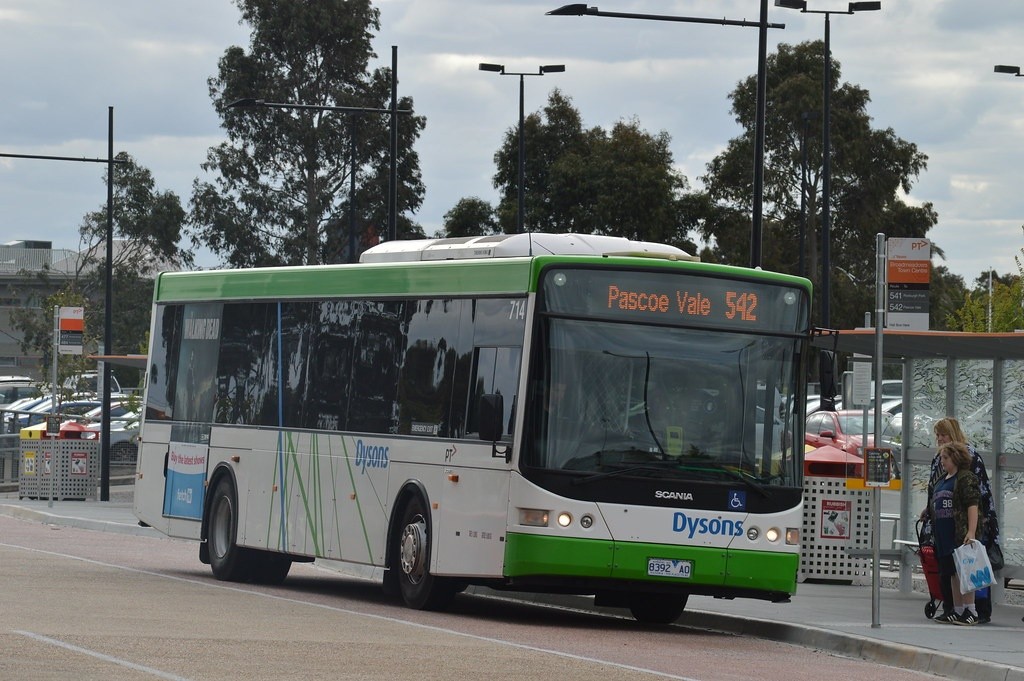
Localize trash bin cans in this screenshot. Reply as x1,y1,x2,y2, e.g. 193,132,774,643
19,420,48,500
39,421,99,501
795,444,873,586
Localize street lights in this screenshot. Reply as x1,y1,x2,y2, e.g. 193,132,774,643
770,0,884,351
546,0,784,270
479,62,570,235
226,41,417,244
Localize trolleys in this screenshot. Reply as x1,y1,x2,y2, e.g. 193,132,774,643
915,513,952,618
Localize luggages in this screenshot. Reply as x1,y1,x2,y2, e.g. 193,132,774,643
916,516,944,618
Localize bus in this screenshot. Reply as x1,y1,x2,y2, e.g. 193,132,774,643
132,232,845,628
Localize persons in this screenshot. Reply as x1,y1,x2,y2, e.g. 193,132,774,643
921,417,1001,623
920,442,981,626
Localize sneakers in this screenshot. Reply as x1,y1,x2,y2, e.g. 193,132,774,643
946,609,978,627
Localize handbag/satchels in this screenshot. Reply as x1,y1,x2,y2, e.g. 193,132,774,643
956,538,998,594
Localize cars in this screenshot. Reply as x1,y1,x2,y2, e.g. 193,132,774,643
1,368,144,464
746,376,904,472
